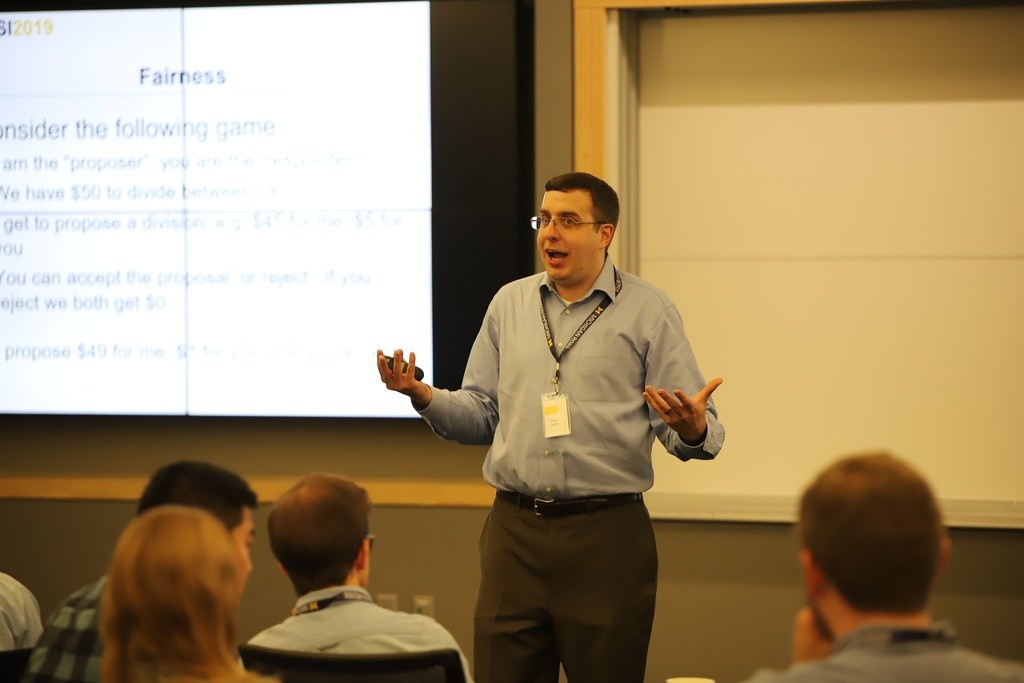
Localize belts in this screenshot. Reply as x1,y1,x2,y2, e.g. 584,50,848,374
495,488,643,518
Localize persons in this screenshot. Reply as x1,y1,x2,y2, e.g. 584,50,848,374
239,472,472,683
99,503,281,683
24,462,256,683
746,453,1023,683
376,170,724,683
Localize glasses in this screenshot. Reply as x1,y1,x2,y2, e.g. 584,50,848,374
530,215,607,231
367,534,376,551
